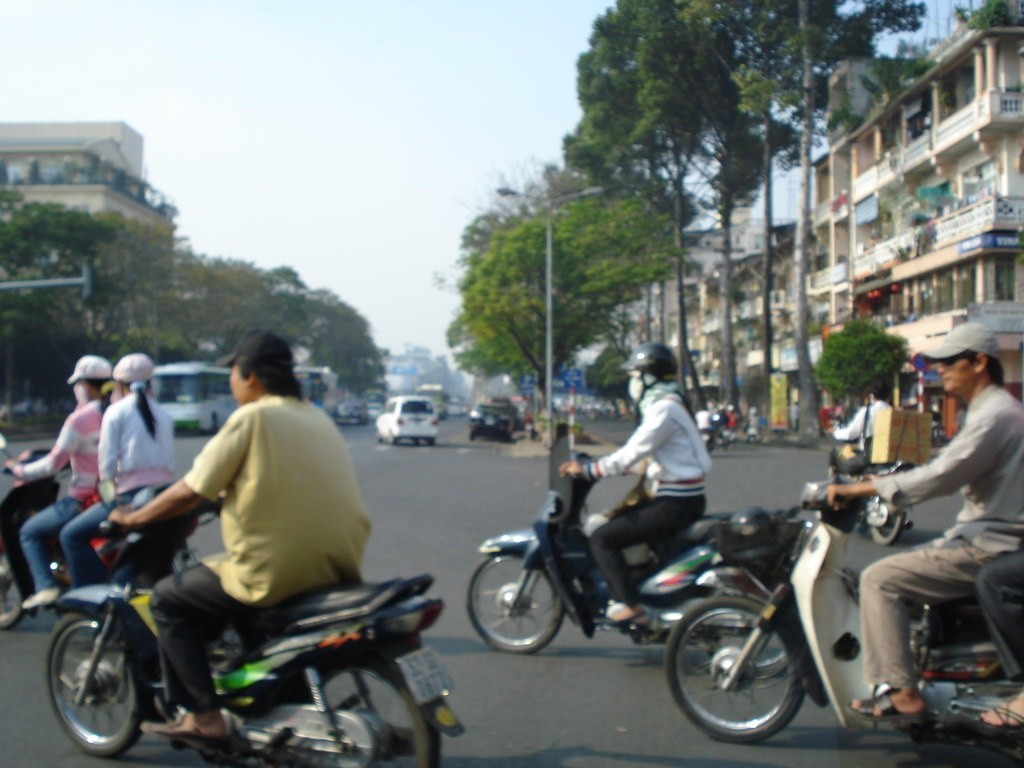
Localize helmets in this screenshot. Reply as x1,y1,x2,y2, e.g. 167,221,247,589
618,342,677,375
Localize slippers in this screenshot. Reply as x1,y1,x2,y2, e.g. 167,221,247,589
845,688,927,724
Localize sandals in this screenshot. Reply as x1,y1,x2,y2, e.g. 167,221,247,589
980,700,1024,734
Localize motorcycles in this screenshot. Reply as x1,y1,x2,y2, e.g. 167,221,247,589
41,479,468,768
463,451,801,679
660,444,1024,759
0,430,193,635
821,410,913,547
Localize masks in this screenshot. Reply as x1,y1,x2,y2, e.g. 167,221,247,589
72,384,88,403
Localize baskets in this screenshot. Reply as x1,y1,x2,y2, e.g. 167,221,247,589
712,511,805,570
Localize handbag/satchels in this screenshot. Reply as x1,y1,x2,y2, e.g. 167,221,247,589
835,443,868,475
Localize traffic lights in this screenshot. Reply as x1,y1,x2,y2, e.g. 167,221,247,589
79,260,108,309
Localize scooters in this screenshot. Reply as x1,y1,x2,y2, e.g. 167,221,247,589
700,410,762,452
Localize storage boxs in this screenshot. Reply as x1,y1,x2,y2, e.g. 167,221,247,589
870,408,932,465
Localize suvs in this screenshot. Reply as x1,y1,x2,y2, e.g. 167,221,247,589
373,395,438,446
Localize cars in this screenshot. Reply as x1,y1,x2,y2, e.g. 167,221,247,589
465,400,515,441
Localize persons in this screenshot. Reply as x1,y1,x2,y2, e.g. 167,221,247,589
108,332,373,746
694,399,763,444
832,381,892,477
976,550,1024,731
794,402,800,432
829,322,1024,720
4,351,176,610
558,342,708,627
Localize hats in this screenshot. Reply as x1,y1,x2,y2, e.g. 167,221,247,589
113,353,153,383
67,355,112,384
924,321,1000,361
215,329,295,369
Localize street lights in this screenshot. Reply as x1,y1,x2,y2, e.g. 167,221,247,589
496,184,606,451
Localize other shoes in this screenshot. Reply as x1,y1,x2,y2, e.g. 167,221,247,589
20,587,60,609
141,713,232,752
592,599,645,625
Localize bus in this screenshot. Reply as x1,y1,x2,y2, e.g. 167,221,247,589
147,361,239,436
338,382,468,426
294,365,339,418
